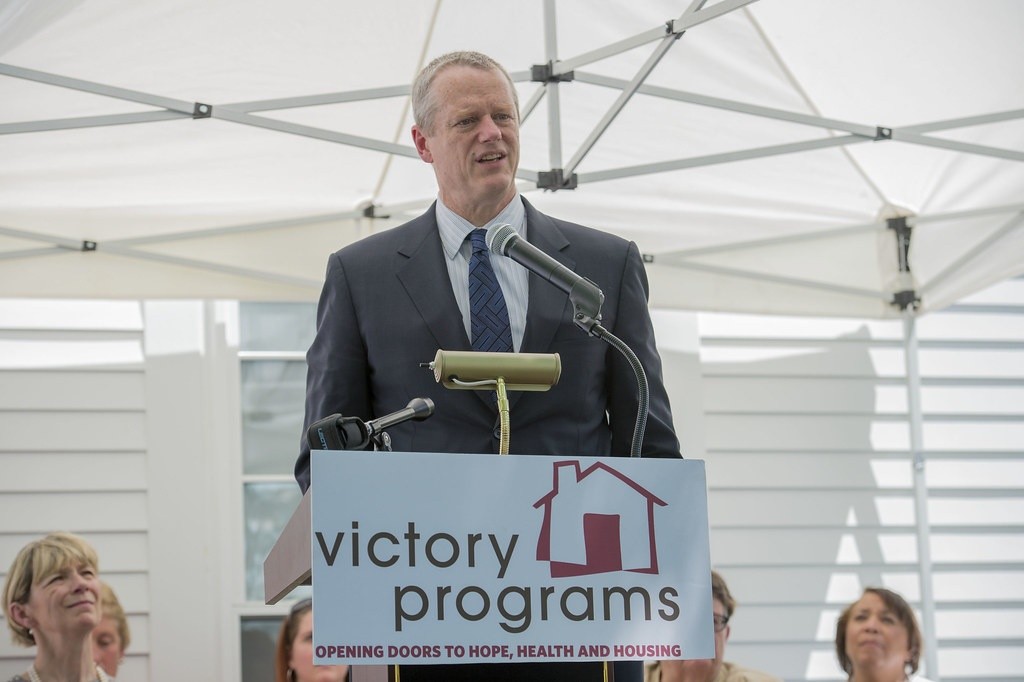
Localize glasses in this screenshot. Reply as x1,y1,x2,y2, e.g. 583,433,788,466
713,612,730,632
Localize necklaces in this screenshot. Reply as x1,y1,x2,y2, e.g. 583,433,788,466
26,662,109,682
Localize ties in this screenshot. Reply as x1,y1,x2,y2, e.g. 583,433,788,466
464,229,515,408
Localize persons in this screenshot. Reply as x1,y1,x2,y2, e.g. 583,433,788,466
91,582,130,677
834,588,934,682
644,570,779,682
275,597,354,682
293,50,686,498
0,533,132,682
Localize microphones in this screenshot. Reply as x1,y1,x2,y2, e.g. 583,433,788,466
307,397,437,451
485,222,605,311
